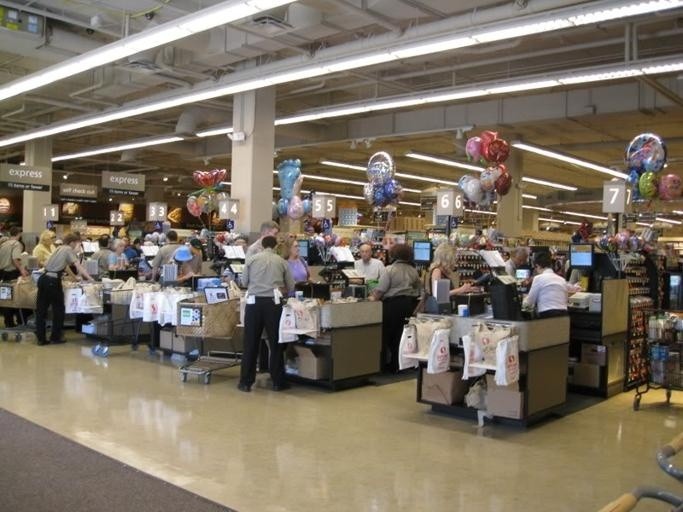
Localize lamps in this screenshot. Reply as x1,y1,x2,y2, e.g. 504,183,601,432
220,140,683,228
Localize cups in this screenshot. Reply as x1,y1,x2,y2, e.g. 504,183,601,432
294,290,303,300
457,304,467,317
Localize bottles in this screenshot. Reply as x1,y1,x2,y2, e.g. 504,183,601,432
648,313,665,339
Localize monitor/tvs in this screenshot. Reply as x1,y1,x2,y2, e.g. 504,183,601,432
569,243,594,269
413,241,431,263
297,240,309,259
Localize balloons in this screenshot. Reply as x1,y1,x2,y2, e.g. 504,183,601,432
273,158,311,220
187,168,230,219
363,151,403,208
458,130,512,209
571,132,682,272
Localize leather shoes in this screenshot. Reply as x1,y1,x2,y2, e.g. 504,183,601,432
272,381,293,392
50,338,67,345
38,340,51,346
237,383,251,392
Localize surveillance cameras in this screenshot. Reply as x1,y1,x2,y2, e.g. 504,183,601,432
145,12,154,20
87,28,94,35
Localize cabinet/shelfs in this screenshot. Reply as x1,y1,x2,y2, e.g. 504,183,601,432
327,242,669,392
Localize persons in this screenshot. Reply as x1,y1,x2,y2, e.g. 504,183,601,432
35,234,94,346
175,239,202,285
503,248,569,317
428,243,481,299
86,234,143,279
0,223,56,328
241,236,291,391
151,231,185,281
368,244,420,377
246,222,309,296
354,243,385,295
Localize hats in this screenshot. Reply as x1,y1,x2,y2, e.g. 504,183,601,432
190,238,203,249
166,230,178,242
175,246,193,262
391,244,413,260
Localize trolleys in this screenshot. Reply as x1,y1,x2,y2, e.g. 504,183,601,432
176,288,260,384
0,270,54,343
80,275,156,358
632,308,682,410
